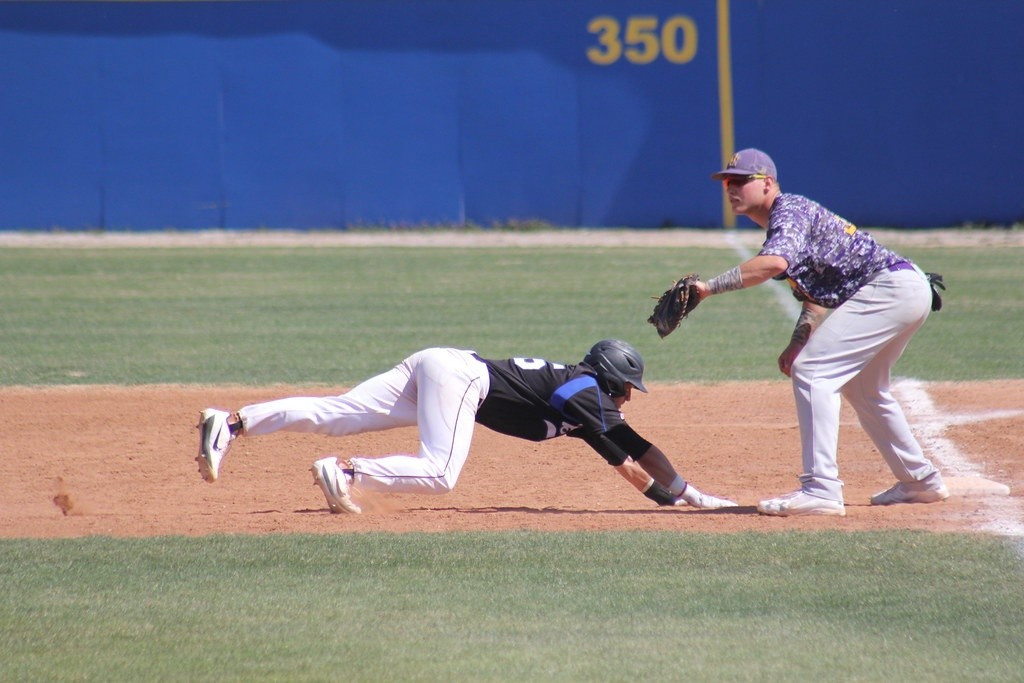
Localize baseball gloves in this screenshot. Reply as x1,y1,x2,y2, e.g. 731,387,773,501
647,273,699,339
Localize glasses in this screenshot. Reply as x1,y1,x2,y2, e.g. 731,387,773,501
723,173,767,189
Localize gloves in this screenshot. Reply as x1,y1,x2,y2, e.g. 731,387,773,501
926,271,946,312
679,482,740,509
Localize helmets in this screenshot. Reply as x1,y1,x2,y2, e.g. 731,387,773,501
584,339,649,399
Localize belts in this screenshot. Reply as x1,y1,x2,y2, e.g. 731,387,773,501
860,263,915,287
472,353,490,405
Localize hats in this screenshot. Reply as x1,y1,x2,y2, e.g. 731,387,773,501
711,147,777,181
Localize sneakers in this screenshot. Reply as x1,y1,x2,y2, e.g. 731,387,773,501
758,481,847,517
195,408,236,484
308,457,363,516
869,471,950,505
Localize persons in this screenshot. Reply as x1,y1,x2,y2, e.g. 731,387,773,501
646,148,950,516
195,340,740,515
